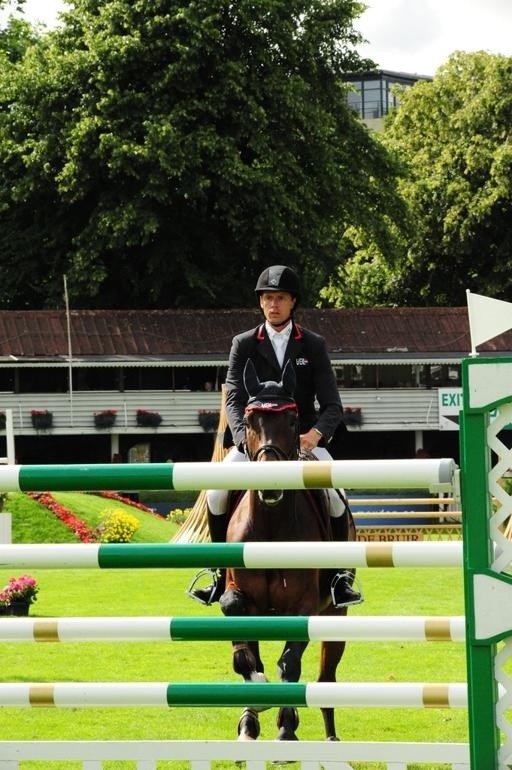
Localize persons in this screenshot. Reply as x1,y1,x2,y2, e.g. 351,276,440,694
192,265,362,602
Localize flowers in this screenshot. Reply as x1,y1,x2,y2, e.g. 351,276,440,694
341,406,365,431
0,573,41,606
0,407,220,439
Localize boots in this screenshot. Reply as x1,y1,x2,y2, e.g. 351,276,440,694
330,509,365,609
191,502,231,604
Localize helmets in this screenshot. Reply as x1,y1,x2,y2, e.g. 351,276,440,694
254,266,300,293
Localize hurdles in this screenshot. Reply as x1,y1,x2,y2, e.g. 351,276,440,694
0,357,511,769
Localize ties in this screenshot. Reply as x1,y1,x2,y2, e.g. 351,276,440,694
272,334,286,370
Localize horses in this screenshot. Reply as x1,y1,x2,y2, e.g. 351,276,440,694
220,356,358,767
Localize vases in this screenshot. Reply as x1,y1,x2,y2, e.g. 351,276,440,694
0,603,29,617
